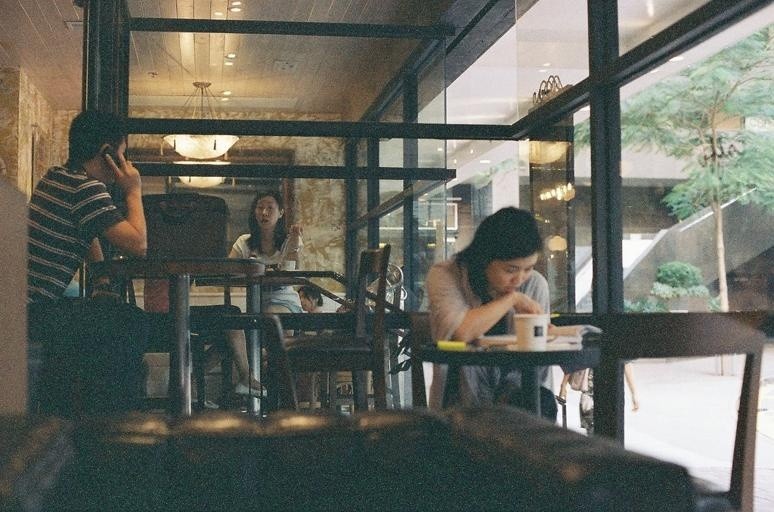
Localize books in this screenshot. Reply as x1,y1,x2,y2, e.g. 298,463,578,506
476,324,602,353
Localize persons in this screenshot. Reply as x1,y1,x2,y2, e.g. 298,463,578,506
28,109,146,413
425,206,558,425
558,361,640,438
189,190,301,416
297,286,332,336
143,195,266,409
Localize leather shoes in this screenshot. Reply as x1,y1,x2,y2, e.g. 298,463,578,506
234,381,268,400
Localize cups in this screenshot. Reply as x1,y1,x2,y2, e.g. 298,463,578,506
276,260,296,271
512,312,549,352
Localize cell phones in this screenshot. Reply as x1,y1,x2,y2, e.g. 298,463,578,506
101,145,120,168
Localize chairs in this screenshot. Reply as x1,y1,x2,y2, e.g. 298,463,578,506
588,306,769,510
408,308,442,416
71,198,391,416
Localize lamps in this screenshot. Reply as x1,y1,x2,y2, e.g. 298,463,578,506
519,141,572,165
173,151,229,188
162,82,239,160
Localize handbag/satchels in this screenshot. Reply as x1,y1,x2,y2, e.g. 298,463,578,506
141,192,230,262
337,263,407,313
